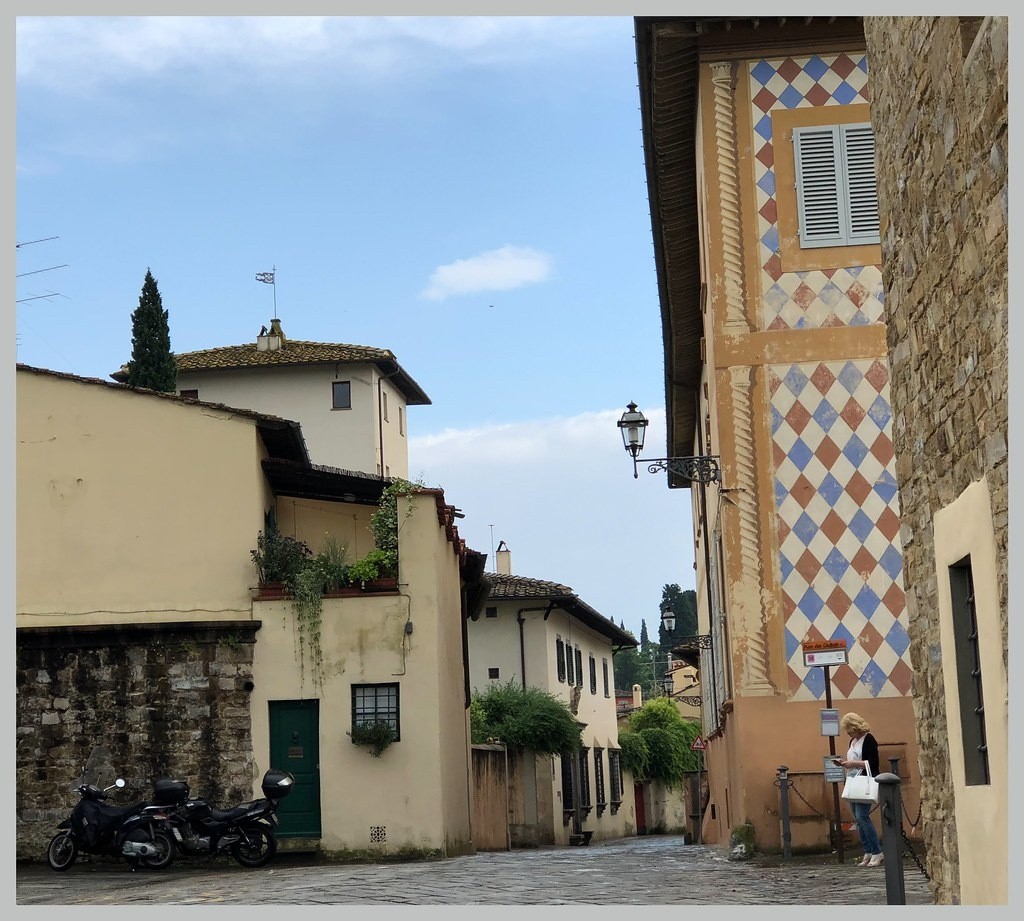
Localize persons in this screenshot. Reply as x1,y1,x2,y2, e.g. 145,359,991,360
833,712,884,868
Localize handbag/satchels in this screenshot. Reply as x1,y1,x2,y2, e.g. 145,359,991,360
841,760,879,804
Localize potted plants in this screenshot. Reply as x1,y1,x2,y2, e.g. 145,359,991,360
247,478,424,696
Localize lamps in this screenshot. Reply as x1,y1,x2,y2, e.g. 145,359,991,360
617,400,722,487
664,605,713,650
777,766,790,781
664,674,702,708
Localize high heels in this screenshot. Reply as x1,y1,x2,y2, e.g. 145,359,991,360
857,852,884,868
